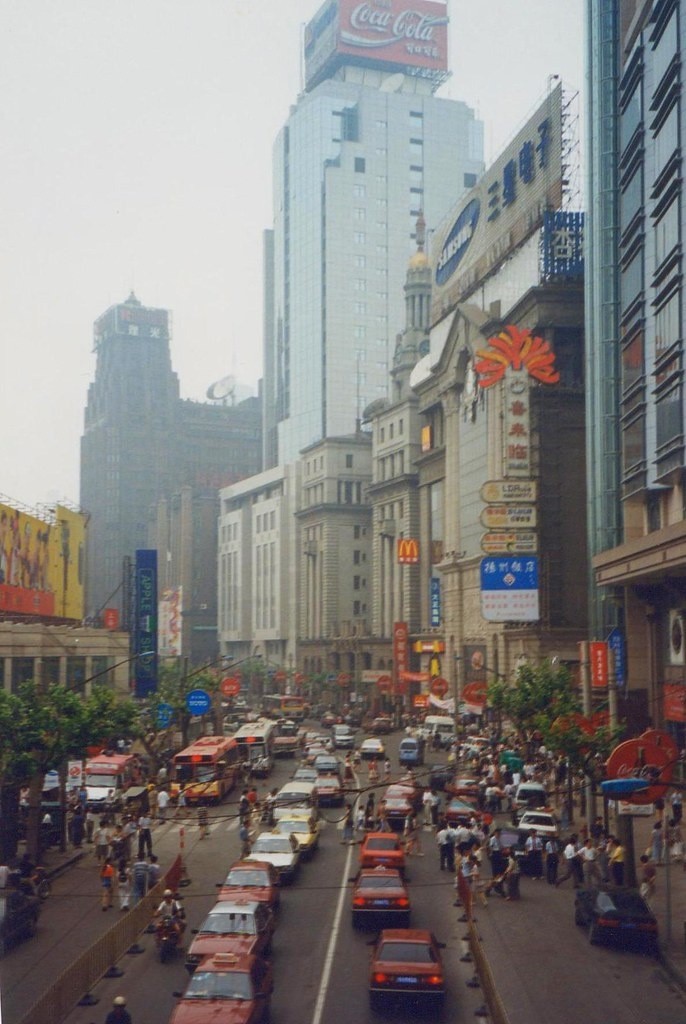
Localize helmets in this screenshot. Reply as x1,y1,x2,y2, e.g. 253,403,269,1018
112,996,126,1007
162,889,172,898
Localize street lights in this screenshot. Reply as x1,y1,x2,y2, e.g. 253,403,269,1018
180,654,236,750
216,652,264,733
56,651,158,852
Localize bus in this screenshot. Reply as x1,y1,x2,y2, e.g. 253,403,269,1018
172,735,241,805
231,721,276,777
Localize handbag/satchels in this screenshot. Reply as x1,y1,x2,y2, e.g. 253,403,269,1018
205,825,210,835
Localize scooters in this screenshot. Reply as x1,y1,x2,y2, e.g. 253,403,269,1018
20,870,54,902
152,910,187,963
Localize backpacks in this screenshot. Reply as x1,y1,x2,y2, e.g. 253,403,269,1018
117,869,127,883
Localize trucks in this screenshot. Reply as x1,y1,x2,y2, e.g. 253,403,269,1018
81,750,136,811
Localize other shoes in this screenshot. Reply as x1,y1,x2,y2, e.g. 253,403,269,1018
108,904,114,908
417,852,425,857
102,906,107,912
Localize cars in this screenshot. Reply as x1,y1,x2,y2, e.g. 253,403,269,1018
0,887,43,957
574,883,659,951
357,832,408,873
216,859,282,915
516,810,561,842
245,828,300,889
359,738,386,760
363,927,447,1014
361,717,392,734
260,692,358,861
346,863,412,928
375,764,484,830
165,951,274,1024
184,898,277,975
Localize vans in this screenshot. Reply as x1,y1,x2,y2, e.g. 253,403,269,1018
422,715,459,745
513,782,552,827
398,738,425,765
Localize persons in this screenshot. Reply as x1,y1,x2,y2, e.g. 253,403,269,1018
105,996,131,1024
1,701,686,940
